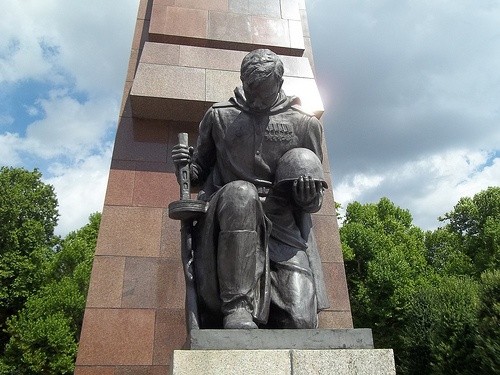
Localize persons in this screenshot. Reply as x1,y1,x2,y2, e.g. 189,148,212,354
168,48,329,330
